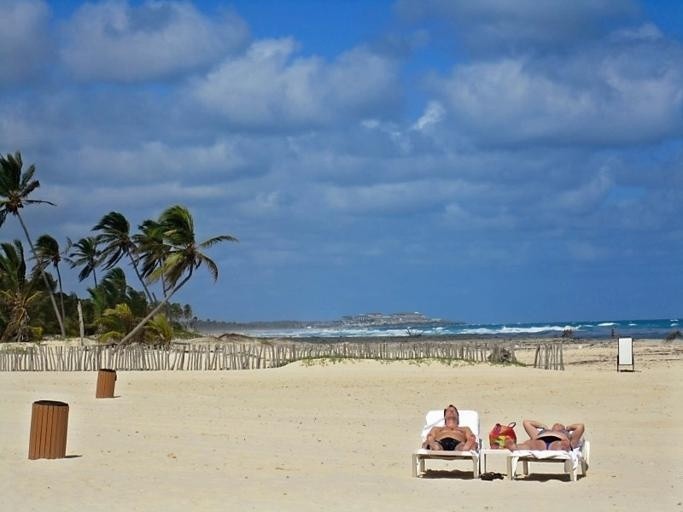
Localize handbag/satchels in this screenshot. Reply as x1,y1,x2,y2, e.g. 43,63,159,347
490,421,516,449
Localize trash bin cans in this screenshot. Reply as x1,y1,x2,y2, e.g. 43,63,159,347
96,369,117,398
28,400,69,460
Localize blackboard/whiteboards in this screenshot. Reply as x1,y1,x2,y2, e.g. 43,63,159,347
618,337,633,365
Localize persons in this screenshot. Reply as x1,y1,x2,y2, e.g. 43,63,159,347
505,420,584,451
421,405,478,451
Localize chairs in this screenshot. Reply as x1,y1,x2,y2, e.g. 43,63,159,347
509,430,590,482
408,403,486,480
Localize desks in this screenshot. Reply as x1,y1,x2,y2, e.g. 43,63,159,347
478,449,511,478
